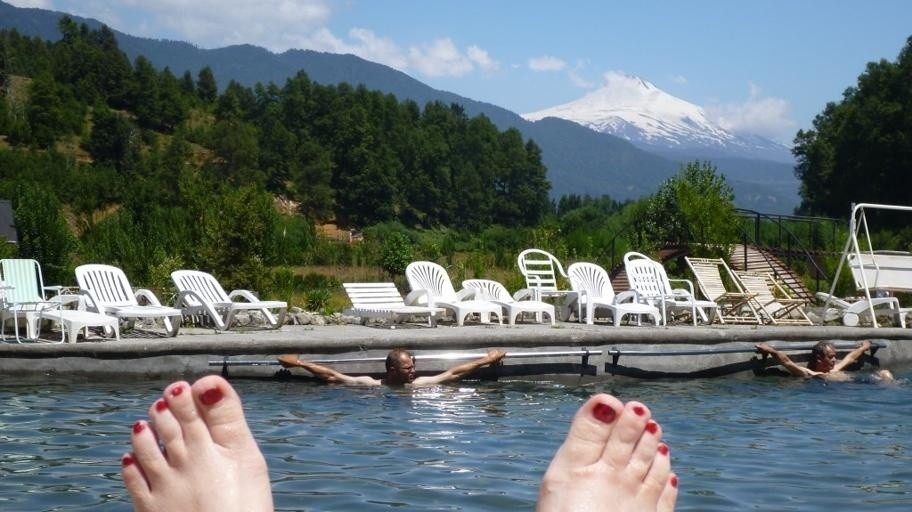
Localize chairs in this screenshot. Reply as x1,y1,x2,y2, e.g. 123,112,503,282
0,253,289,347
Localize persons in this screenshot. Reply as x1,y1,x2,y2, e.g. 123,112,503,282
276,348,509,390
753,341,896,384
119,372,679,511
